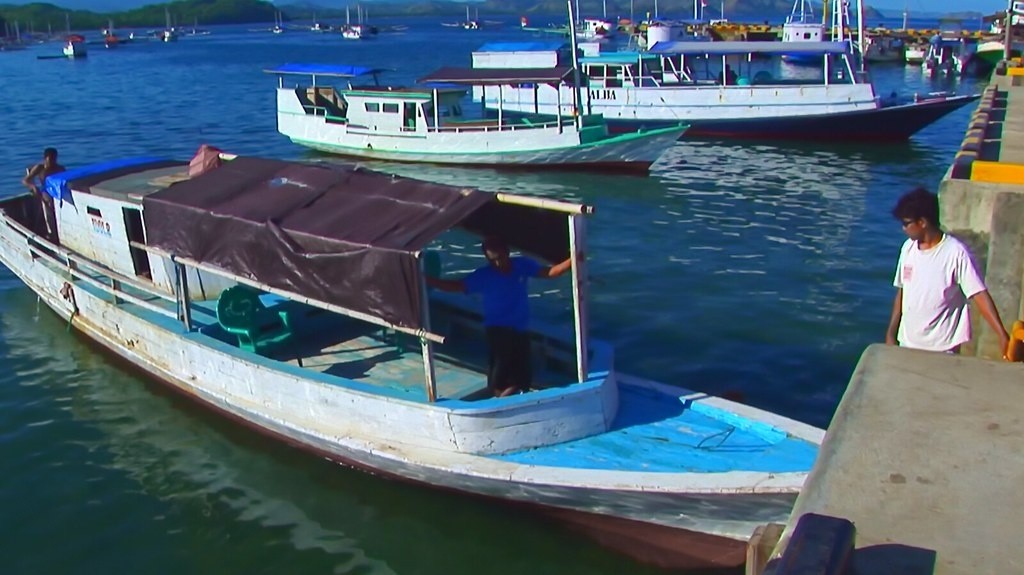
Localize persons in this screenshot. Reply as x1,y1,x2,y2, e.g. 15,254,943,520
886,189,1011,360
91,219,111,236
422,235,572,396
404,103,408,126
23,147,66,245
718,64,737,85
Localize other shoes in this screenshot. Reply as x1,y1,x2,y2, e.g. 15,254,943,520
500,386,517,397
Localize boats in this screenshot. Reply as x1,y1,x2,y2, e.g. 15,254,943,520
568,17,614,39
262,62,691,174
340,4,371,41
63,39,86,57
470,38,980,144
147,28,211,38
463,6,478,30
780,0,824,64
0,141,828,575
272,11,282,34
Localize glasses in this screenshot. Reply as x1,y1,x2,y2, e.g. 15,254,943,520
902,219,920,227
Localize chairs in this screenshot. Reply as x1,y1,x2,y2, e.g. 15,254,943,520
379,251,447,352
735,75,750,85
215,284,303,368
